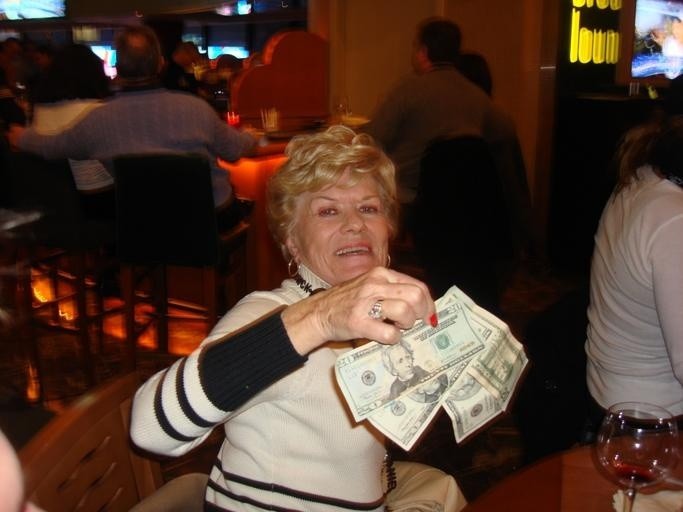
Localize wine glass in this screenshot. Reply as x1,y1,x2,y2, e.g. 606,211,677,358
594,400,677,511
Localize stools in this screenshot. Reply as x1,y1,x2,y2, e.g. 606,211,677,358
109,148,249,369
2,144,138,386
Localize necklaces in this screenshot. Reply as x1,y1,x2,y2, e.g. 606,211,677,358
288,267,326,297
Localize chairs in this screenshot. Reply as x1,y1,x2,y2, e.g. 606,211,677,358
12,366,228,512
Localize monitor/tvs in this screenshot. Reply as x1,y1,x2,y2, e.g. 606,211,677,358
87,41,118,80
180,33,252,67
0,0,67,22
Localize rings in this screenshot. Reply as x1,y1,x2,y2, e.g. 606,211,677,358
369,302,382,319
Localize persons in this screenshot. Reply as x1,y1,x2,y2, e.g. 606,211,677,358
381,340,430,398
353,14,501,265
418,377,447,402
584,72,683,429
0,432,45,512
128,123,439,512
1,23,264,313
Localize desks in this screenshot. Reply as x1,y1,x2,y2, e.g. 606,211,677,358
3,206,47,401
